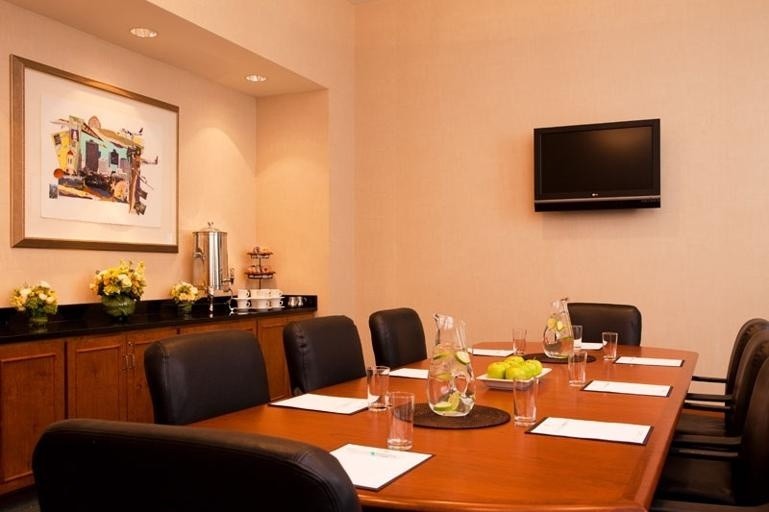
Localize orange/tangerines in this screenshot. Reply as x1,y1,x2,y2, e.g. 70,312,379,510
488,356,543,379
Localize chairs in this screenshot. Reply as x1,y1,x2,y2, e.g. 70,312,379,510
672,328,769,433
685,319,769,406
141,330,270,425
369,307,427,372
647,354,767,511
566,302,642,344
31,418,363,511
283,314,366,395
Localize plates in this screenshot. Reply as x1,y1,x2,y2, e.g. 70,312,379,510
250,297,286,300
233,307,251,311
232,297,252,300
476,367,553,391
251,306,285,312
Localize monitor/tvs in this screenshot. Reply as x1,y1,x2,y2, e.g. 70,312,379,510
532,119,661,211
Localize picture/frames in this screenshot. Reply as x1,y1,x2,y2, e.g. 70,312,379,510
9,53,181,251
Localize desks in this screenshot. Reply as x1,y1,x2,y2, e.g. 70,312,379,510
181,341,699,512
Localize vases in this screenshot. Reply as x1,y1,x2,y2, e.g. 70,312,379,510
176,302,192,315
27,313,47,332
105,295,135,316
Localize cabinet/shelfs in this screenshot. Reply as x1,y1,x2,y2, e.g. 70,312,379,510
257,312,315,401
179,320,258,339
65,329,179,422
0,339,66,495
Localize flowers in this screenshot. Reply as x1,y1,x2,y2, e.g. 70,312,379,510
8,281,58,316
90,257,148,302
167,280,200,301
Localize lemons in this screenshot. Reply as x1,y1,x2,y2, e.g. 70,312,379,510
547,318,569,336
431,346,472,415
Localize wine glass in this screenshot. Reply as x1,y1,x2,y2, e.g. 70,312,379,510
250,289,284,298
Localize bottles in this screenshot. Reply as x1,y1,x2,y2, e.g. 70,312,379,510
542,297,575,359
426,312,475,417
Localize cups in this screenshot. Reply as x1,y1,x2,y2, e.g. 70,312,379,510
602,332,618,361
385,391,415,450
573,325,583,357
236,289,250,299
513,327,527,356
567,350,588,387
366,366,391,412
251,300,284,307
288,295,308,309
512,375,536,427
